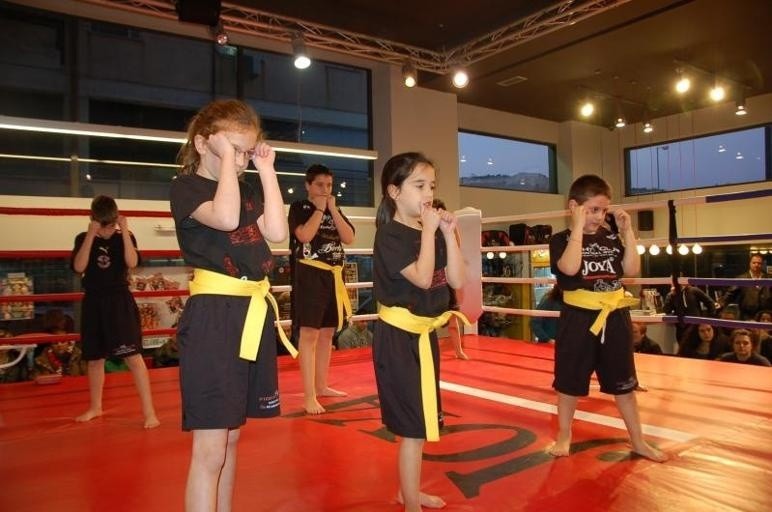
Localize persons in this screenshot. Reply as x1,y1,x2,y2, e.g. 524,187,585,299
286,166,357,416
544,173,671,463
371,151,465,512
168,97,281,512
427,199,469,362
71,195,162,428
630,253,771,366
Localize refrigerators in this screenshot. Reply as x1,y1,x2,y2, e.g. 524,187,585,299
500,248,558,342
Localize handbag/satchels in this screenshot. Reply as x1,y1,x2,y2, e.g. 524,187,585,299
482,230,509,245
510,223,552,245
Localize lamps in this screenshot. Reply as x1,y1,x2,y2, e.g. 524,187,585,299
676,66,748,117
210,25,471,89
637,242,703,256
582,98,654,133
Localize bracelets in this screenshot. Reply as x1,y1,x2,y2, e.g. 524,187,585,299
315,208,324,213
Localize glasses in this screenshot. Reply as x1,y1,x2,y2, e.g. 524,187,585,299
232,145,256,160
